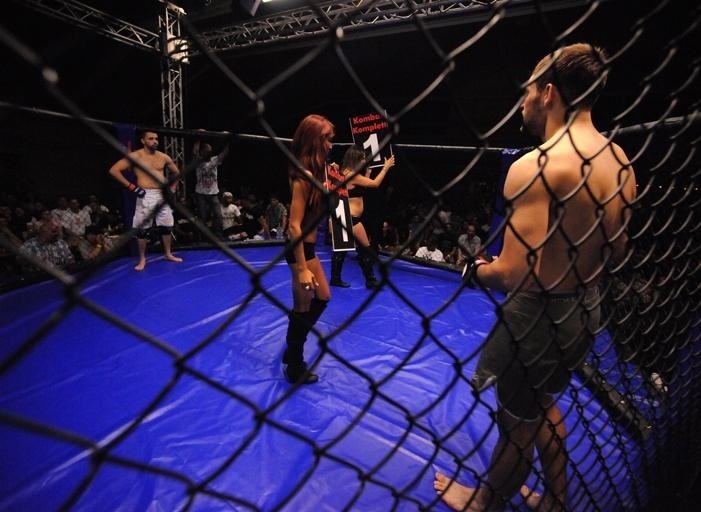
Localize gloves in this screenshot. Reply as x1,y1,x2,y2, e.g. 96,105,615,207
169,183,176,194
462,254,498,290
127,182,146,198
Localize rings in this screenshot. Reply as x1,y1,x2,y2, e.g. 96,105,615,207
305,285,311,291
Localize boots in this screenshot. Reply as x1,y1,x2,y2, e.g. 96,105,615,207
282,295,328,384
329,251,350,288
357,245,381,289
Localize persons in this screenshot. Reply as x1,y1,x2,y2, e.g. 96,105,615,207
108,130,181,269
601,189,699,393
377,189,497,266
283,115,354,383
432,44,637,510
1,184,291,292
190,128,233,236
329,144,396,289
